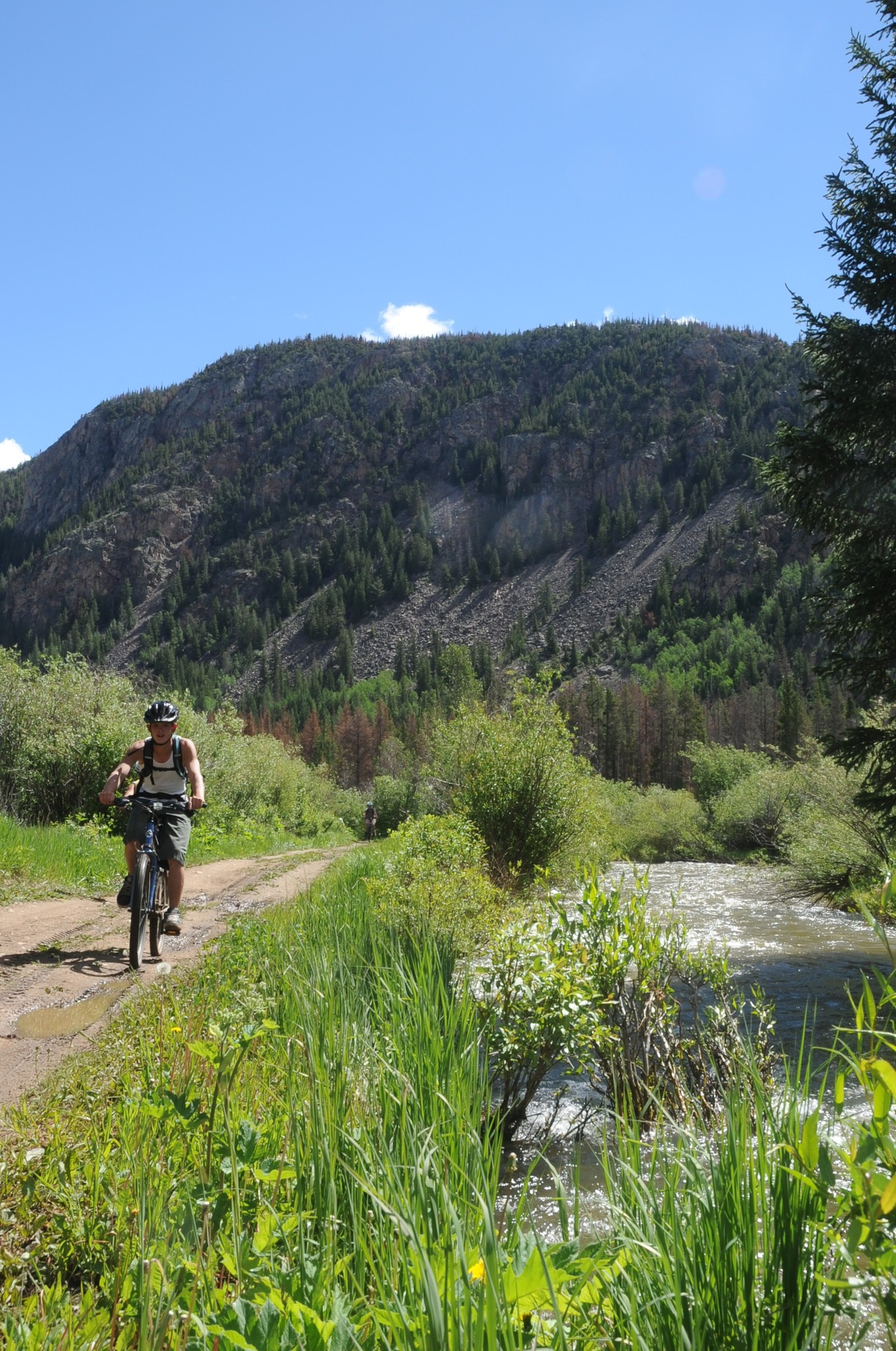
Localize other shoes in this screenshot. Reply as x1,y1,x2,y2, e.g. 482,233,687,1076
364,832,368,836
373,830,377,836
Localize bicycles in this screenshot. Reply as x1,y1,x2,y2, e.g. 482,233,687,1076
111,795,208,970
364,816,379,843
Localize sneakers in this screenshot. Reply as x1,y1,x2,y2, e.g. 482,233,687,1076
163,907,182,931
117,872,134,908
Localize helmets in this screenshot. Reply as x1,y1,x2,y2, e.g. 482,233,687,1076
366,802,373,807
143,700,180,724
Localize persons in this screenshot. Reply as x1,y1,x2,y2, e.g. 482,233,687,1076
100,699,204,941
117,760,144,809
363,801,378,837
